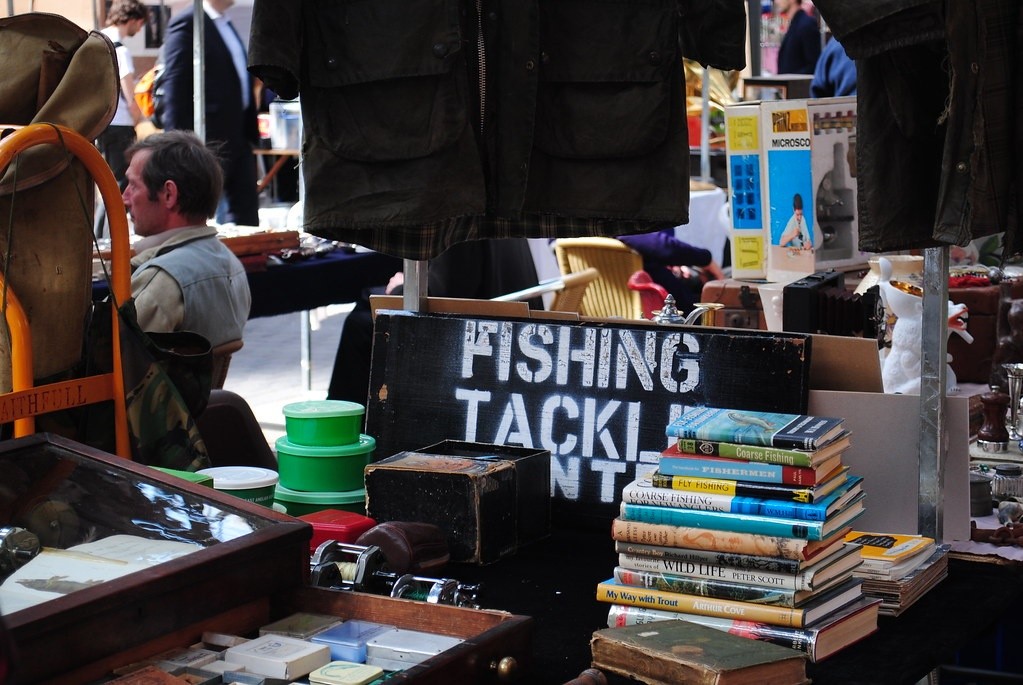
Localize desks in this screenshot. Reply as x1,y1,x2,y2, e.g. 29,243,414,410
252,148,300,193
91,248,404,391
700,268,1023,383
527,185,727,311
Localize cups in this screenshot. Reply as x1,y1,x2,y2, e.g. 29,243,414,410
257,114,271,140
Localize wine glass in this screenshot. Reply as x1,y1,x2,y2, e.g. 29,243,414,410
1001,362,1023,441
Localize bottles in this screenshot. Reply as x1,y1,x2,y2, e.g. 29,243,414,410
269,97,302,152
991,464,1023,500
977,385,1009,452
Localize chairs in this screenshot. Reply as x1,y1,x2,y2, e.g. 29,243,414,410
555,236,644,319
628,271,669,321
490,267,599,313
211,339,243,390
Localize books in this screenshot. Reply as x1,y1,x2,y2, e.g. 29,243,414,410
592,407,951,685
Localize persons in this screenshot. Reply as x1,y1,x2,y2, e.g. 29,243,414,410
778,193,813,251
809,35,857,97
615,227,724,325
93,131,251,391
97,0,149,215
163,0,260,226
771,0,821,100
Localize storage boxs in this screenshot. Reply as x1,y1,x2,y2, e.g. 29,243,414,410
760,97,858,283
58,585,533,685
196,400,377,517
365,438,551,566
724,98,782,282
0,431,313,685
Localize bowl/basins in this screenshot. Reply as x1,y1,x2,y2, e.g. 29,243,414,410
197,396,377,518
